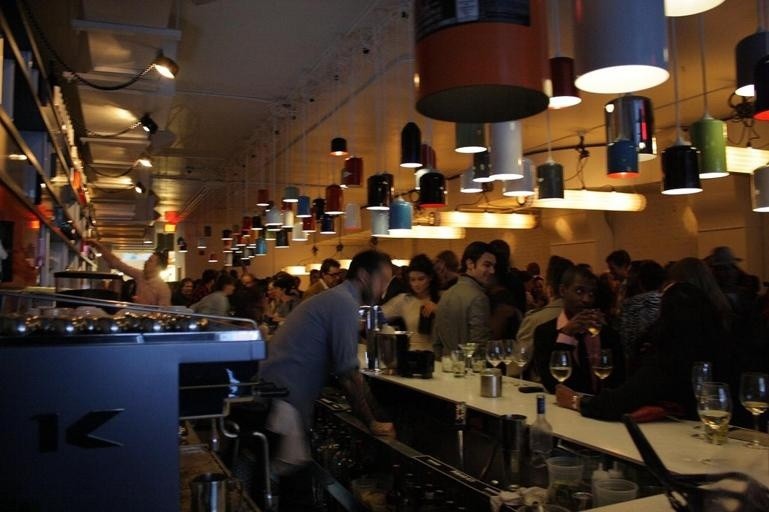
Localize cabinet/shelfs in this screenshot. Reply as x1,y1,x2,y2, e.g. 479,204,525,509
0,1,100,287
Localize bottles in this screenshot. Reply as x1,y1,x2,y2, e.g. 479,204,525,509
527,392,551,482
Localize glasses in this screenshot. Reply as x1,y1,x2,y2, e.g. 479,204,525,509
326,271,342,277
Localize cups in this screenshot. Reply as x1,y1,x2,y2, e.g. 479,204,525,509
594,474,639,506
480,374,502,398
188,473,244,511
449,351,468,383
544,452,586,499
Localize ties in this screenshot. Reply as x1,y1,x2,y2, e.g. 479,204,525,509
575,335,590,372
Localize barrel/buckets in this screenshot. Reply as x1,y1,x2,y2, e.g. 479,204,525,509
373,328,415,373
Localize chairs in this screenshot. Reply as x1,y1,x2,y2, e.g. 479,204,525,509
217,361,320,511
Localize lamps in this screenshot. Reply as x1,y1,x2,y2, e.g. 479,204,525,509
88,183,147,195
72,54,181,91
82,112,158,139
85,152,155,178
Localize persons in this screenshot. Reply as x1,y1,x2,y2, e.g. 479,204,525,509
255,249,397,509
172,239,768,422
83,234,171,308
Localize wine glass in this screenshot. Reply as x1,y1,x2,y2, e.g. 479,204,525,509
689,361,710,402
738,373,769,452
487,338,501,370
513,342,529,382
592,349,613,396
549,348,574,391
461,342,478,377
697,381,729,470
501,340,516,379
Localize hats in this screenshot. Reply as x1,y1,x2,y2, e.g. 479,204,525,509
269,273,294,288
